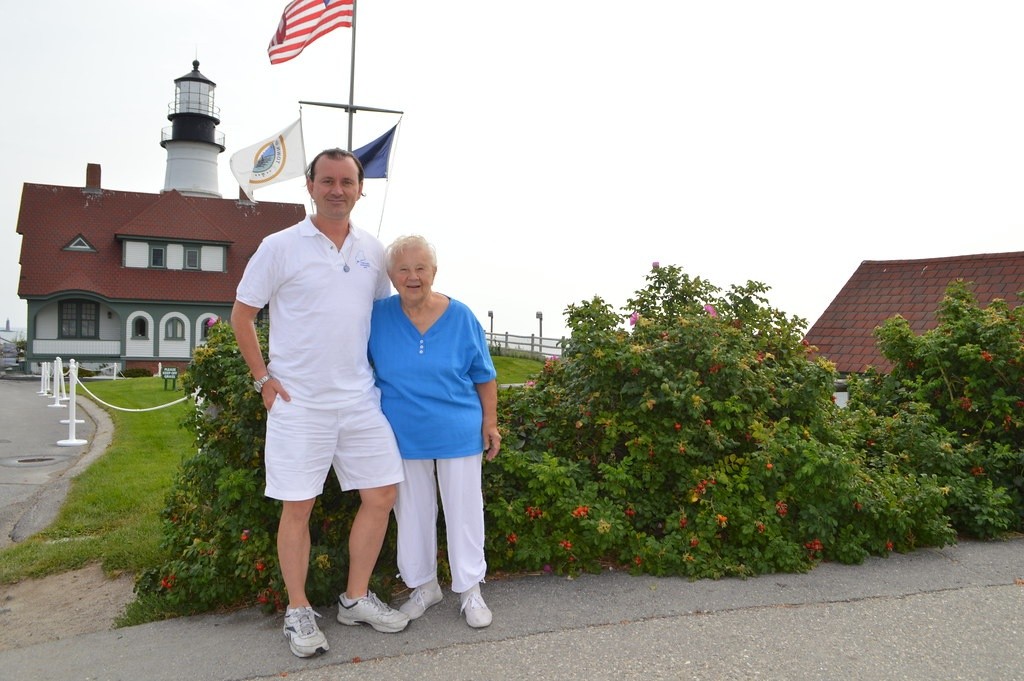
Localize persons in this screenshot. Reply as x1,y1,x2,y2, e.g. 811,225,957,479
367,236,503,628
230,147,410,657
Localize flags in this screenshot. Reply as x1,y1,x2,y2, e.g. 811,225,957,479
266,0,353,65
229,118,307,203
353,122,399,178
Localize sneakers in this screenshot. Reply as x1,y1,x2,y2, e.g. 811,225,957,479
399,585,444,620
336,589,409,633
282,605,330,658
458,583,493,628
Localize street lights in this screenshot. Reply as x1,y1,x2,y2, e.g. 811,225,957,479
488,311,494,345
536,311,542,353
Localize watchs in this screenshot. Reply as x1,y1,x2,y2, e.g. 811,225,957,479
254,374,271,393
